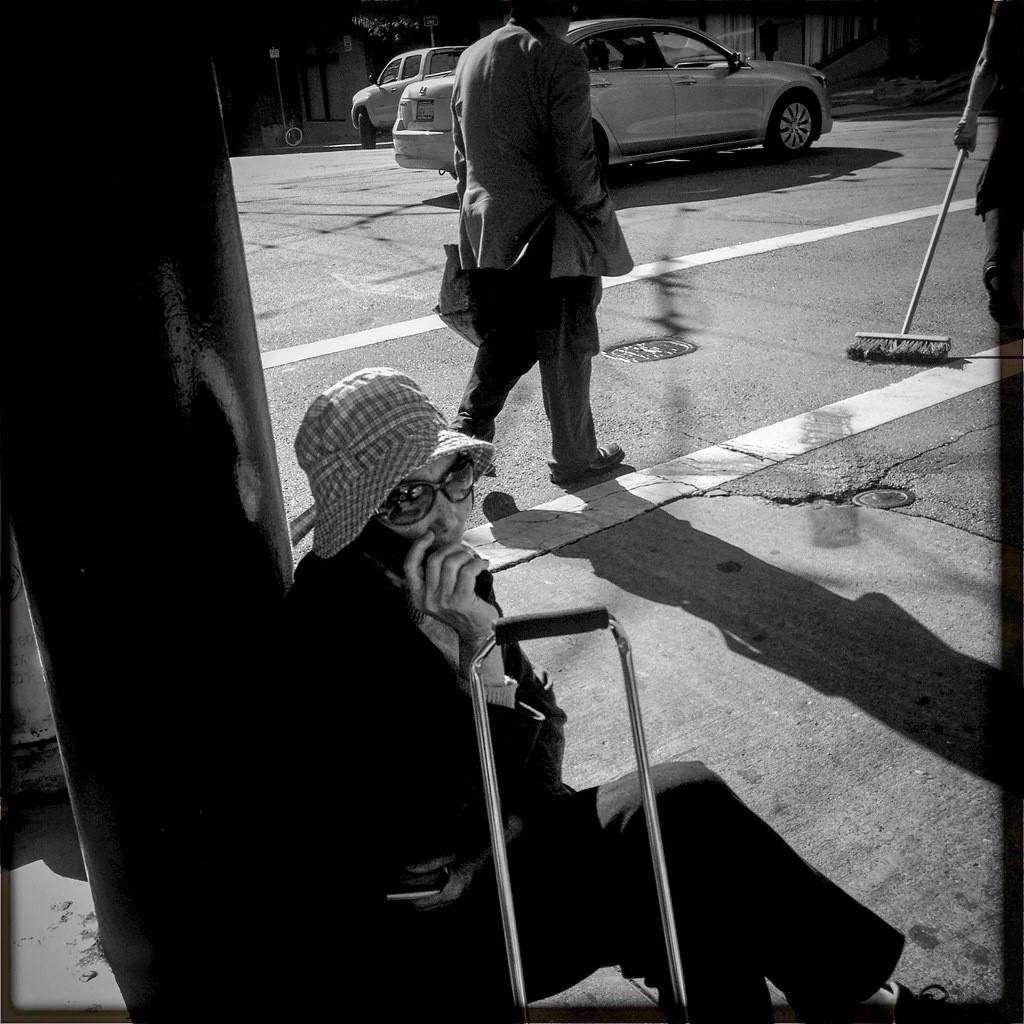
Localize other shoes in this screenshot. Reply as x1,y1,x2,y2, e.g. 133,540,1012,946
882,982,1007,1024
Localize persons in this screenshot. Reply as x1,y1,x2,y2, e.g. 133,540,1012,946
263,367,1004,1024
448,1,635,481
954,0,1024,324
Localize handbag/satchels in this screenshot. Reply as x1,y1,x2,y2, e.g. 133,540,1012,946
431,244,510,347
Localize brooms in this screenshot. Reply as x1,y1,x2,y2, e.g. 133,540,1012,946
845,150,970,367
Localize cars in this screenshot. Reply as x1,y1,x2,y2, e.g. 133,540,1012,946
351,45,469,150
392,17,834,181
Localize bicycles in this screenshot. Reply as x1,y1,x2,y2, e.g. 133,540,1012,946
284,103,303,147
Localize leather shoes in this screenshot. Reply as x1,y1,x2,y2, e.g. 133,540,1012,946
548,443,625,482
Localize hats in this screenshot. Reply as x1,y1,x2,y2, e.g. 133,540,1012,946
294,367,495,560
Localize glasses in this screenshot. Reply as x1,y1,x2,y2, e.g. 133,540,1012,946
373,450,474,526
606,49,610,54
